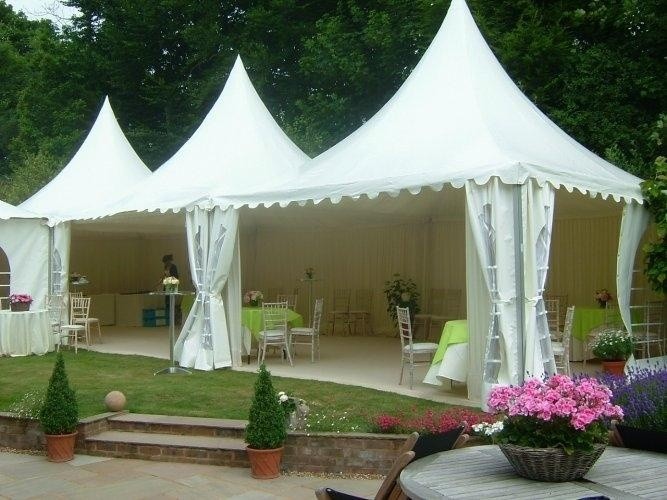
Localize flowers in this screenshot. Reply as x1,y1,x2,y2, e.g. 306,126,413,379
562,359,666,433
587,321,636,359
4,284,35,308
481,370,628,450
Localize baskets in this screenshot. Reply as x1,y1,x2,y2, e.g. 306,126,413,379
11,301,30,311
496,440,604,482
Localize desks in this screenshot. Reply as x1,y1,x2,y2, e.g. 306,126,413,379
87,290,118,329
0,308,53,359
399,443,665,500
152,289,196,381
116,285,175,329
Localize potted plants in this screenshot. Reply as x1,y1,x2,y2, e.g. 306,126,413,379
244,360,290,479
34,342,81,466
385,273,417,337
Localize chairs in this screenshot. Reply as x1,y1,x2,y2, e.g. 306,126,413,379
313,426,464,499
395,286,467,394
40,288,100,359
542,287,665,376
238,289,372,365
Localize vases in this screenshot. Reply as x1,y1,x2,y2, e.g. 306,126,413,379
602,361,628,379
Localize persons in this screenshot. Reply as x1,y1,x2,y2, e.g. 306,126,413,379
160,253,182,328
